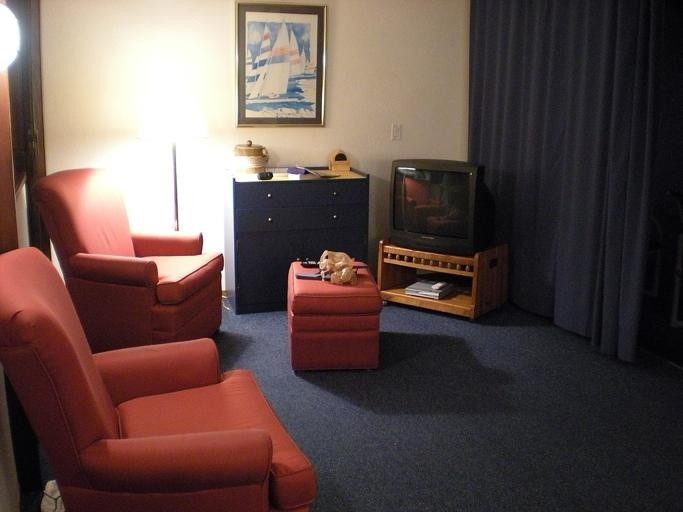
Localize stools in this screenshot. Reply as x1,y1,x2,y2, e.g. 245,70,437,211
287,259,382,371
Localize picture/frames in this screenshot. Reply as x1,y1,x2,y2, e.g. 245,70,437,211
236,3,328,128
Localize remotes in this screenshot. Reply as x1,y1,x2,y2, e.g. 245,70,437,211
431,281,449,291
300,261,321,268
296,272,331,282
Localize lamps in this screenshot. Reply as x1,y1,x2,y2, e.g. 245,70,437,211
0,5,21,70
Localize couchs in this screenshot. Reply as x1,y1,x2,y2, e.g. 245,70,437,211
0,249,317,512
36,166,224,355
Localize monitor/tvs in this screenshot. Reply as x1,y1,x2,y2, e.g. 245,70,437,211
386,158,487,258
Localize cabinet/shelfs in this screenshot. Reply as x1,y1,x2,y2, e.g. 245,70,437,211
378,239,511,323
223,166,371,315
